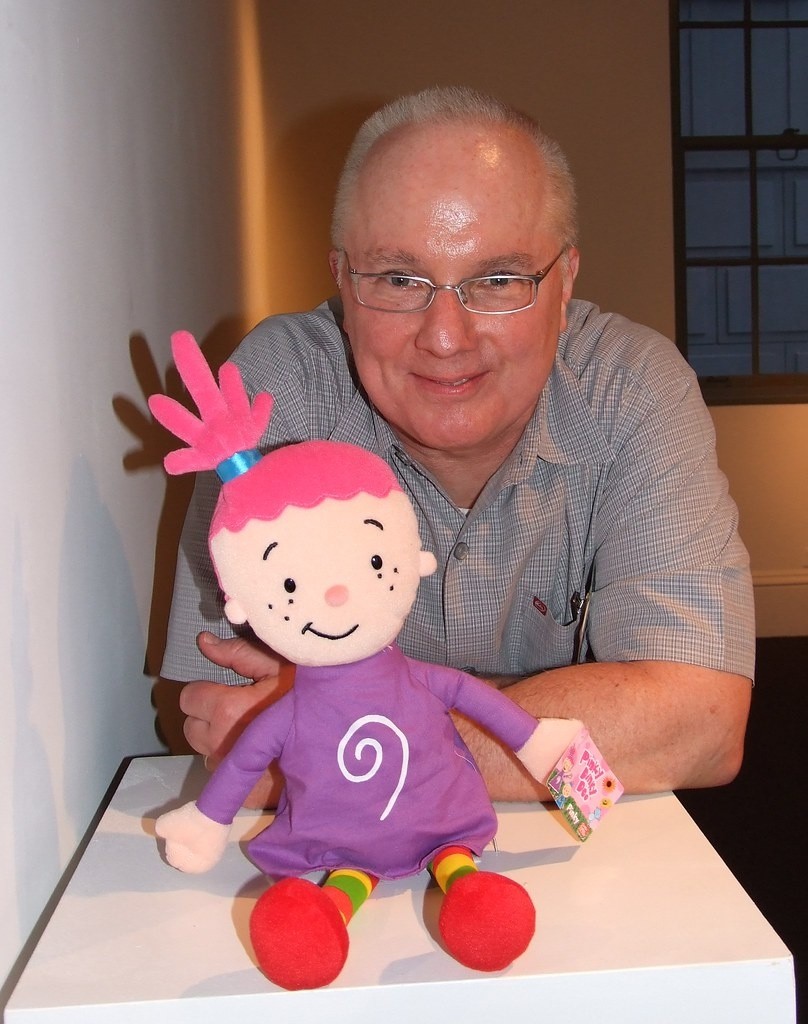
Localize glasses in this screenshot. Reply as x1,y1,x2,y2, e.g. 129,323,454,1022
344,240,572,314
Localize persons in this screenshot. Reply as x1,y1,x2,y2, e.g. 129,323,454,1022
161,88,755,811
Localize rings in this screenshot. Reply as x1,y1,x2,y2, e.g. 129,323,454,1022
204,755,208,768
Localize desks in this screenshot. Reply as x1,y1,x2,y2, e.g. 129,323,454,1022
3,755,797,1024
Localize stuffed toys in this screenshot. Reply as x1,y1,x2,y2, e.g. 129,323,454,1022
147,332,584,990
